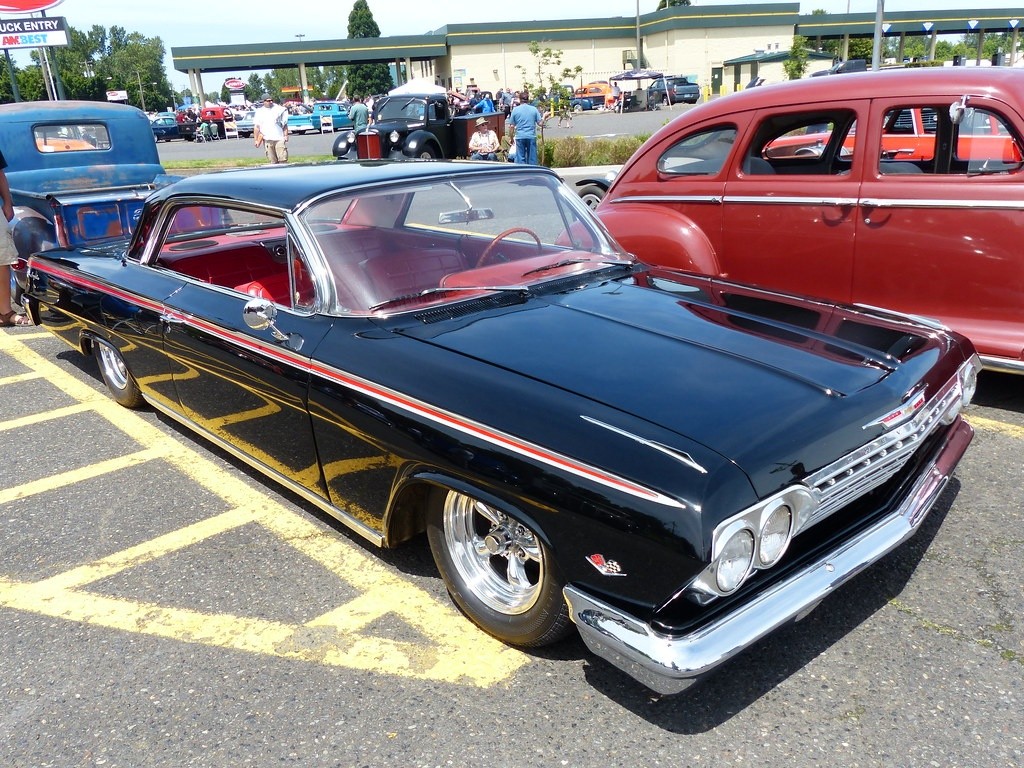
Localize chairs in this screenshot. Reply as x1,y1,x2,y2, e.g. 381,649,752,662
224,120,238,138
195,126,212,143
320,114,334,134
210,124,220,141
172,246,276,289
235,267,376,313
361,249,461,304
295,230,393,273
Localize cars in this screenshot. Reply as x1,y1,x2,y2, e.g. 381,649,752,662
0,100,226,308
556,58,1023,377
23,160,983,700
148,111,182,143
559,82,619,114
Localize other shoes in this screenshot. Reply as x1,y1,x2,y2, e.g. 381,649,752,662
558,124,561,129
565,125,571,129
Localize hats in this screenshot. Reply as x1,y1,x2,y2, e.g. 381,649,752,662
261,93,271,101
486,94,489,98
354,95,361,99
474,117,488,128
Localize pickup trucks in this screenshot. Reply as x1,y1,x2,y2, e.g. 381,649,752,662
647,77,701,106
286,102,354,134
178,107,233,141
332,93,506,161
234,111,256,138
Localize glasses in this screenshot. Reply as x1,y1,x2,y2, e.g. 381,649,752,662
482,123,487,126
265,100,273,102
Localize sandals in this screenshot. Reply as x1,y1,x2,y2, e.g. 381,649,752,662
0,310,33,326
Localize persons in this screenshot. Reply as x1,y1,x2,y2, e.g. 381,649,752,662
193,118,218,143
447,88,521,118
176,100,264,122
509,91,552,165
289,103,315,116
558,93,573,128
253,93,288,164
609,81,620,104
469,117,499,161
0,150,33,327
346,95,372,132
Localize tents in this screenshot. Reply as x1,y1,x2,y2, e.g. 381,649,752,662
604,69,673,113
